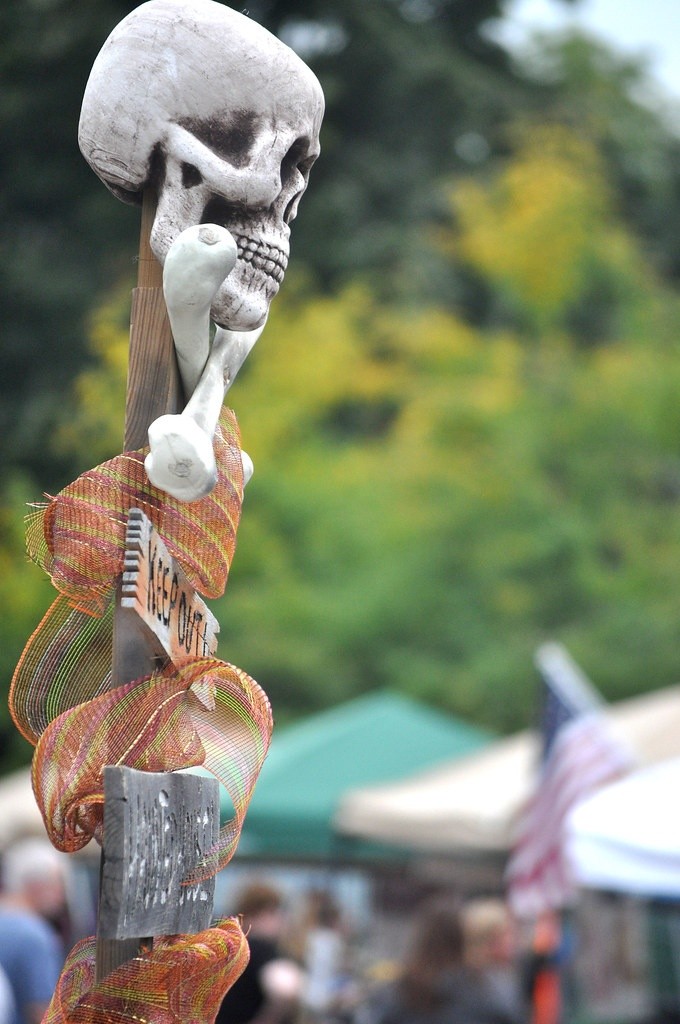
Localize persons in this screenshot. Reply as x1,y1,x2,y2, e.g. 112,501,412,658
0,837,680,1024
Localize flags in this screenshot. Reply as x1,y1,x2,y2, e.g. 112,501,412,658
484,647,648,916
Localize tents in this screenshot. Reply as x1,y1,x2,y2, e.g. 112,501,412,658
0,685,679,897
